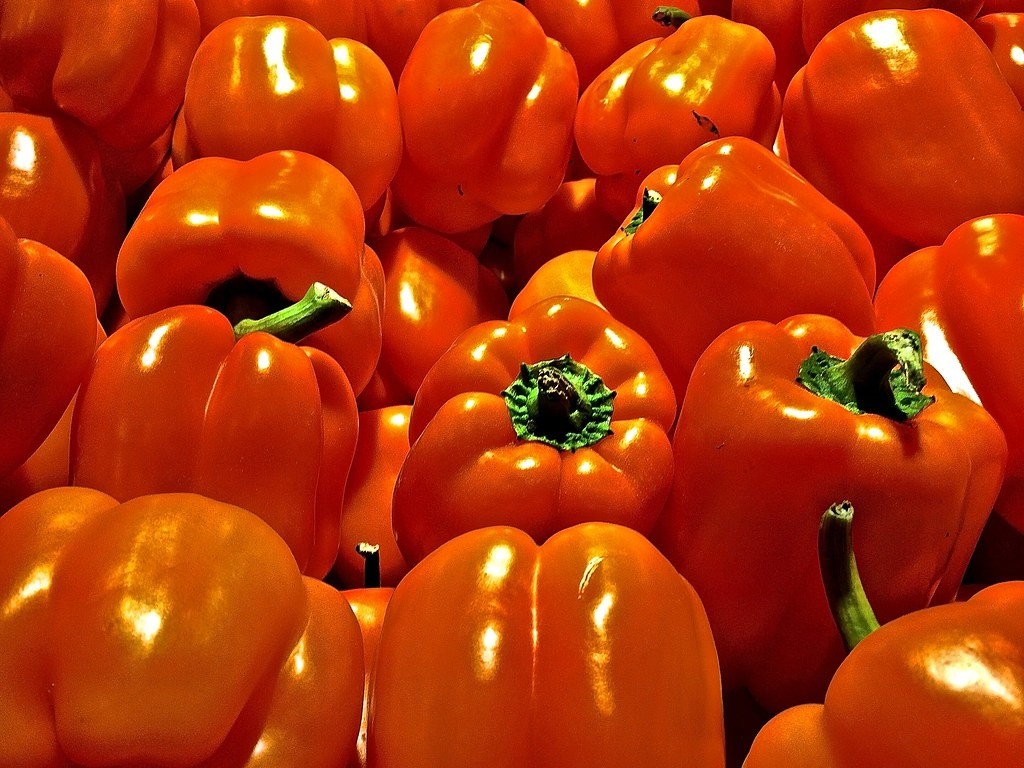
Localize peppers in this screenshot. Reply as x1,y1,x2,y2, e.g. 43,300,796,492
1,0,1024,768
